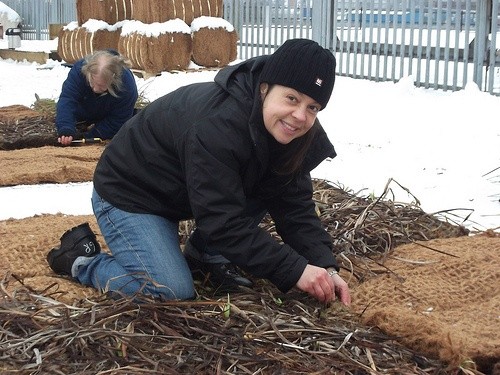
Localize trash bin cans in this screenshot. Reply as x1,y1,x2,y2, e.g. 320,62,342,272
6,27,22,49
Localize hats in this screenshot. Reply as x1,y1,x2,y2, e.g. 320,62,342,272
260,39,336,111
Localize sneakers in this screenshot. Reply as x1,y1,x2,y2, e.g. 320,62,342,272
47,221,100,275
185,253,254,290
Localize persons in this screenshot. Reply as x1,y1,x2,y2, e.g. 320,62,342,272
54,48,138,145
47,38,350,307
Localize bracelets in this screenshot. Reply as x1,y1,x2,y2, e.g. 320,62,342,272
329,271,338,277
